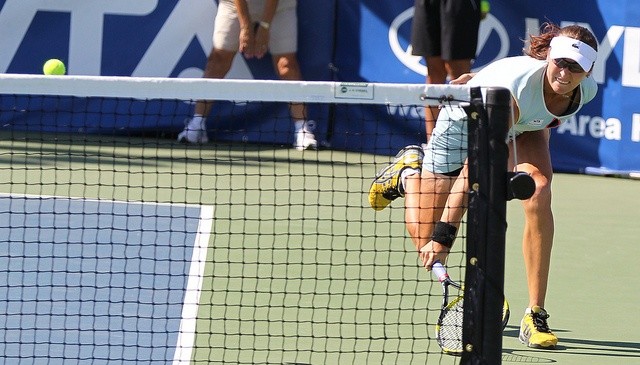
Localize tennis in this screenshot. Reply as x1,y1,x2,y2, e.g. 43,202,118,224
43,59,65,75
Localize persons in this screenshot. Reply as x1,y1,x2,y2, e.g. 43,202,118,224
368,24,597,350
410,0,481,144
177,0,321,152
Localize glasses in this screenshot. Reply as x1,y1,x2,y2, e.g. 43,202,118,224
554,60,582,73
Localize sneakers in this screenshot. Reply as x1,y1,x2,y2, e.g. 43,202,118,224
518,304,559,350
177,125,209,144
293,121,319,150
369,145,424,211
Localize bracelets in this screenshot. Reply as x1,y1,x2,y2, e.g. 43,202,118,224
258,19,271,29
430,219,457,248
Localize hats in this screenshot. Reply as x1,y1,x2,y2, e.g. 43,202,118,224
550,38,597,73
